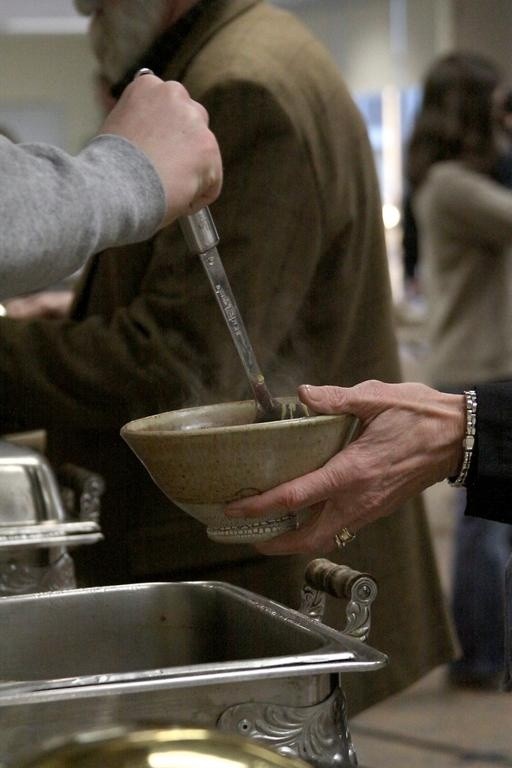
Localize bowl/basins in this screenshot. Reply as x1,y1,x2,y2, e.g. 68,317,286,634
120,396,361,545
